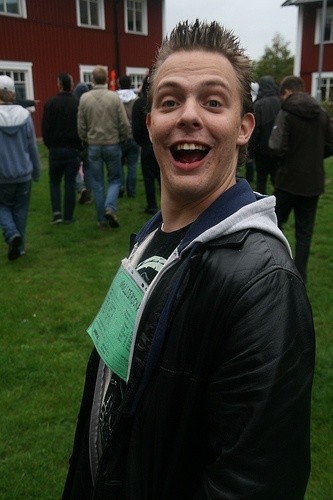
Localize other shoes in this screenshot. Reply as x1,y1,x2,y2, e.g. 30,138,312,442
105,210,120,229
52,215,63,224
8,236,23,260
119,188,124,197
65,219,74,223
79,188,88,204
131,192,138,198
146,206,158,214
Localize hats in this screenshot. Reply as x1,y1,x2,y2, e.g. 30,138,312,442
0,75,15,93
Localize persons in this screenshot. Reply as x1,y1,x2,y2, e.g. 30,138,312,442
62,19,317,500
41,68,161,229
245,76,283,194
0,75,41,261
267,76,333,285
16,99,41,108
